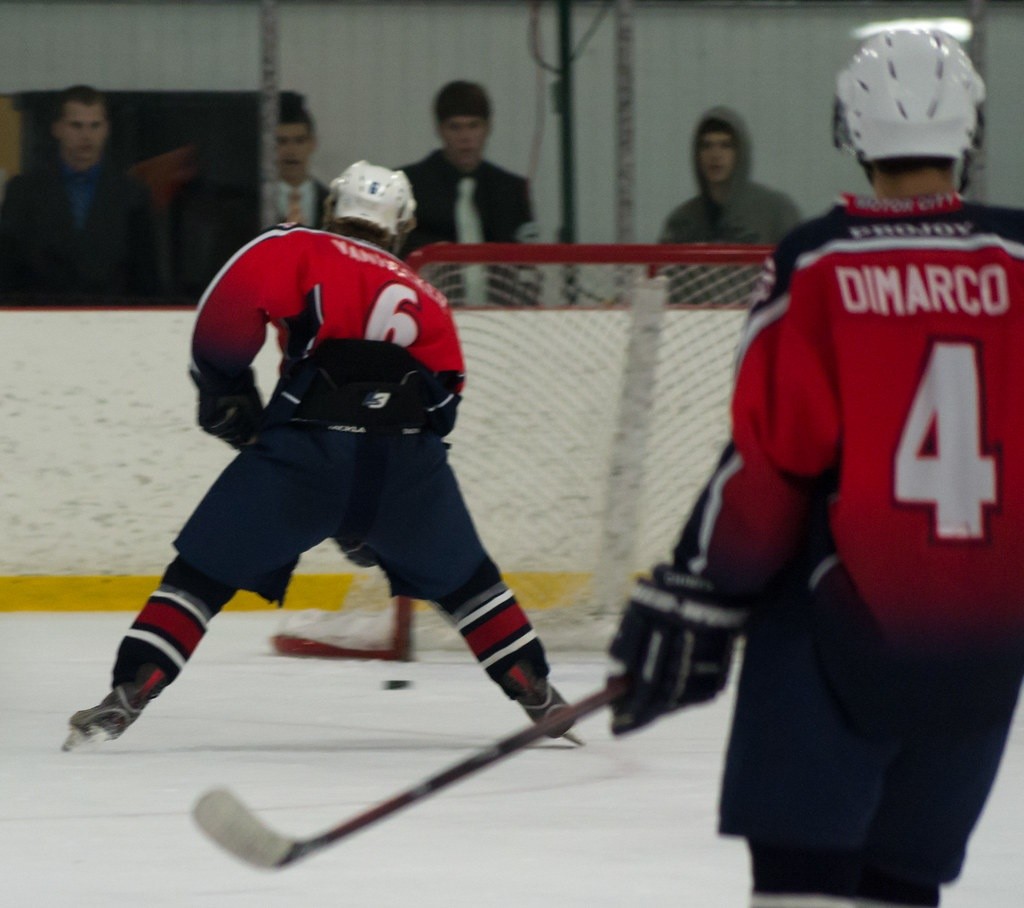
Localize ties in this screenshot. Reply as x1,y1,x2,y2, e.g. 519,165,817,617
288,184,303,223
454,177,488,305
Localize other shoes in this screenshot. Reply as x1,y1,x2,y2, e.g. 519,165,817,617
498,663,590,748
56,664,166,751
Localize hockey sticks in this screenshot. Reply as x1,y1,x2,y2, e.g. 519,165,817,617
188,679,626,869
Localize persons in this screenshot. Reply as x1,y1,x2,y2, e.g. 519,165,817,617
655,103,803,309
393,81,546,308
0,82,155,307
211,92,333,281
64,162,589,755
602,26,1023,908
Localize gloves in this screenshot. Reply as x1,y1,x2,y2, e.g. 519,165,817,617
608,561,748,735
186,367,264,448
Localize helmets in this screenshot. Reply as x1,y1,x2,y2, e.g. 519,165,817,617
321,159,417,236
829,31,988,169
